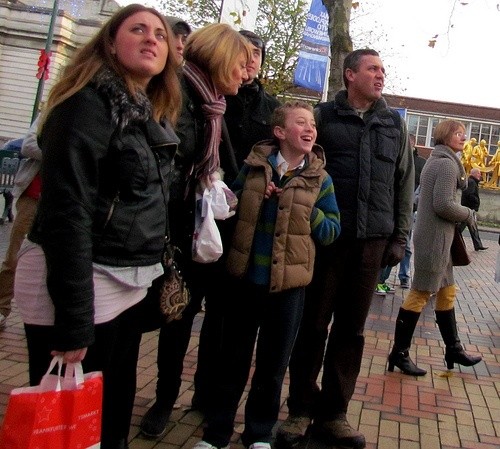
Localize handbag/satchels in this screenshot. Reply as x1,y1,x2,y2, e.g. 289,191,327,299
0,353,104,448
191,180,236,263
140,266,192,333
450,227,471,266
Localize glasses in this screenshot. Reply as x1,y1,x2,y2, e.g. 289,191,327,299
453,132,467,138
244,35,264,49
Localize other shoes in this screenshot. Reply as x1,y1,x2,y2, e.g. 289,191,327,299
249,441,270,449
140,404,170,436
192,441,232,449
475,247,487,251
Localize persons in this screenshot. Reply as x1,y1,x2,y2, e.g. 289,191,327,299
453,170,489,251
460,137,500,192
386,117,481,376
0,0,433,449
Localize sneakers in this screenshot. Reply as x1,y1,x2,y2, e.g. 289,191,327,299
313,413,365,448
276,415,311,445
382,283,396,293
400,278,409,288
374,284,387,295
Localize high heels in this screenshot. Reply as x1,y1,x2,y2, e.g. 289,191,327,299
388,307,427,376
435,306,482,369
